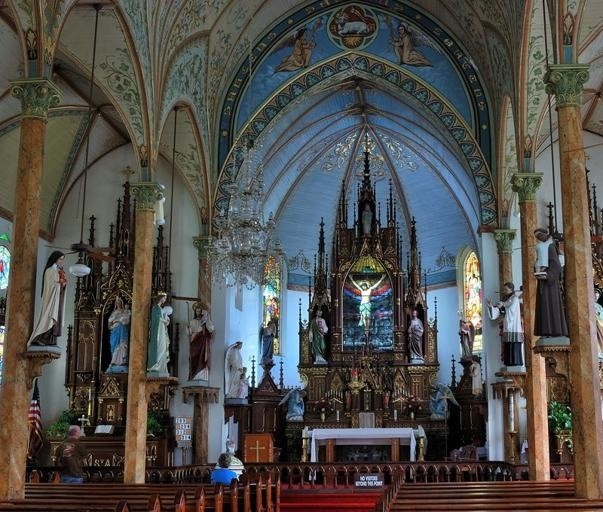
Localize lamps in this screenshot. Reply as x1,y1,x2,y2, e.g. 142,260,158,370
204,1,280,293
65,3,102,277
160,106,178,315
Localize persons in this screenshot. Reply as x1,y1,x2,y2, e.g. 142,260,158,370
272,27,317,75
28,250,68,346
459,320,473,357
408,310,424,360
211,453,237,485
106,296,132,366
348,274,387,336
593,288,603,362
533,227,568,339
263,321,276,361
54,425,90,484
145,291,173,373
224,340,250,398
468,355,484,399
485,282,524,366
278,387,307,421
309,309,329,362
184,298,215,381
390,23,434,68
428,382,460,420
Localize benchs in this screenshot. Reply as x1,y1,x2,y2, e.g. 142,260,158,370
0,475,284,511
373,470,602,511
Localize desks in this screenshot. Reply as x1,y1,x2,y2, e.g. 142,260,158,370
298,425,427,462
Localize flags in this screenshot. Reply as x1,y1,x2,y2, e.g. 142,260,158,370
27,379,43,464
